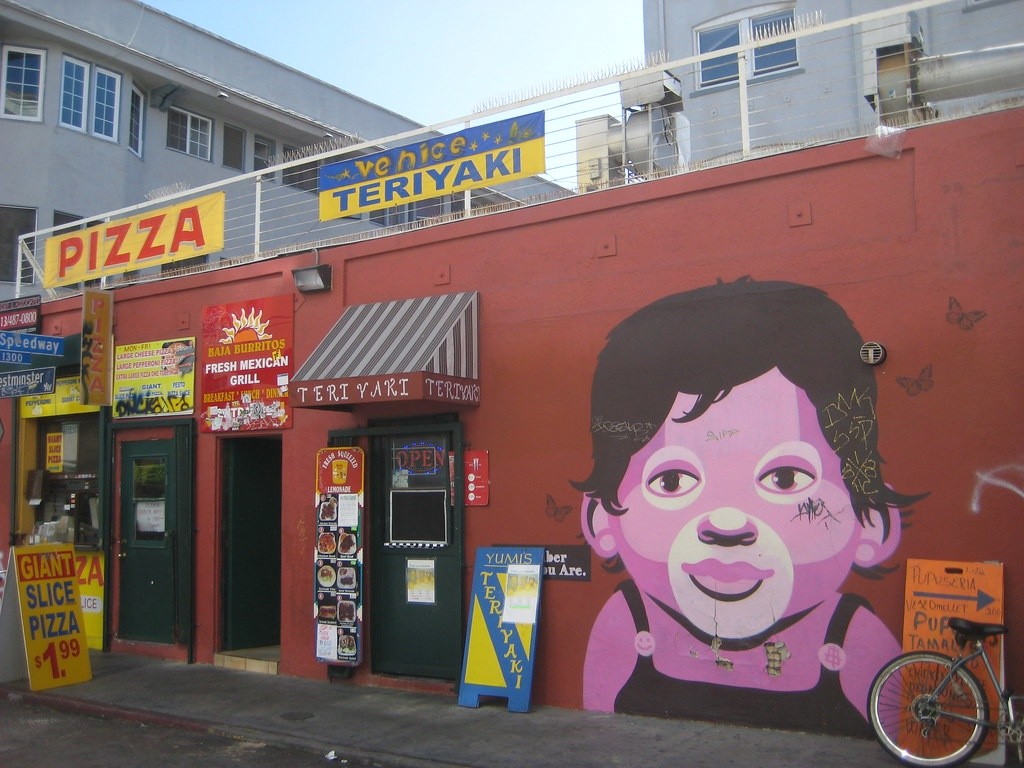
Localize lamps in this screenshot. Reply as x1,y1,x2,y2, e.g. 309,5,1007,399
291,263,334,294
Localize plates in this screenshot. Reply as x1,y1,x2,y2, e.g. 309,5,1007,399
320,502,337,521
338,533,356,554
317,565,336,588
337,567,356,589
337,601,356,622
318,533,336,554
337,635,357,656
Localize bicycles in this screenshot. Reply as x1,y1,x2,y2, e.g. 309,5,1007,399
865,618,1024,768
